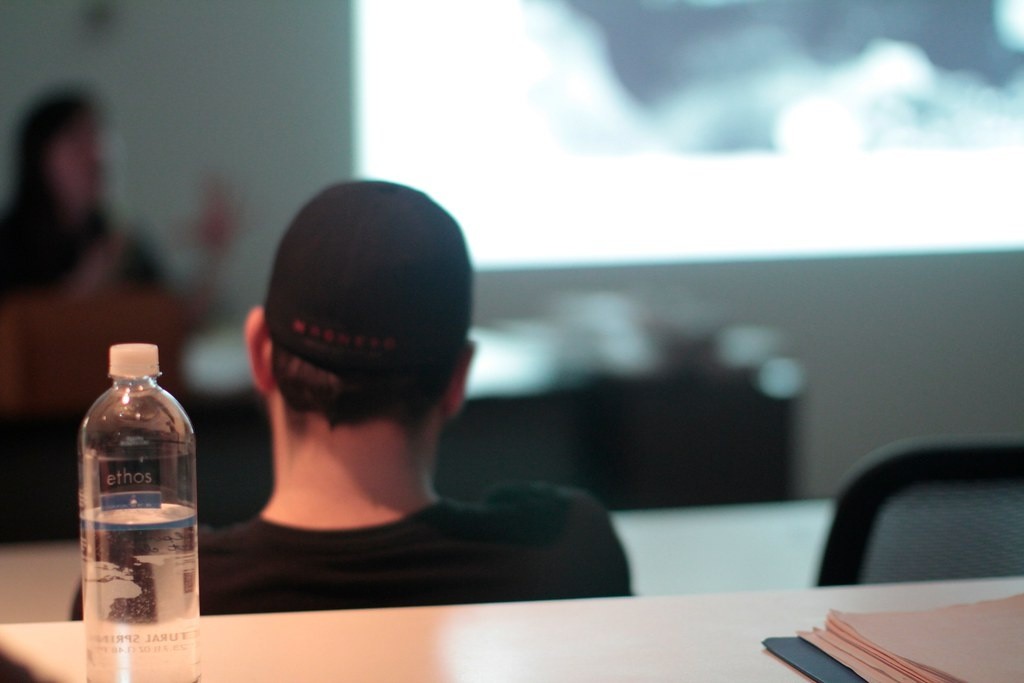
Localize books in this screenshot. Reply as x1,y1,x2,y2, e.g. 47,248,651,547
763,593,1024,683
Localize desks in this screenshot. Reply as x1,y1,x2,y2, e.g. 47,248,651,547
0,576,1024,683
0,499,838,624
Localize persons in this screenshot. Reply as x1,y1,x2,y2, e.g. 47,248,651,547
70,180,631,621
0,90,231,549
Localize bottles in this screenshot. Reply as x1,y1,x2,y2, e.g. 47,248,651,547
77,343,202,683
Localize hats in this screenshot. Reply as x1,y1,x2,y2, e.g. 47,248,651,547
262,182,473,378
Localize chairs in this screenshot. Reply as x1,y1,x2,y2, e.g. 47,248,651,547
817,433,1024,587
